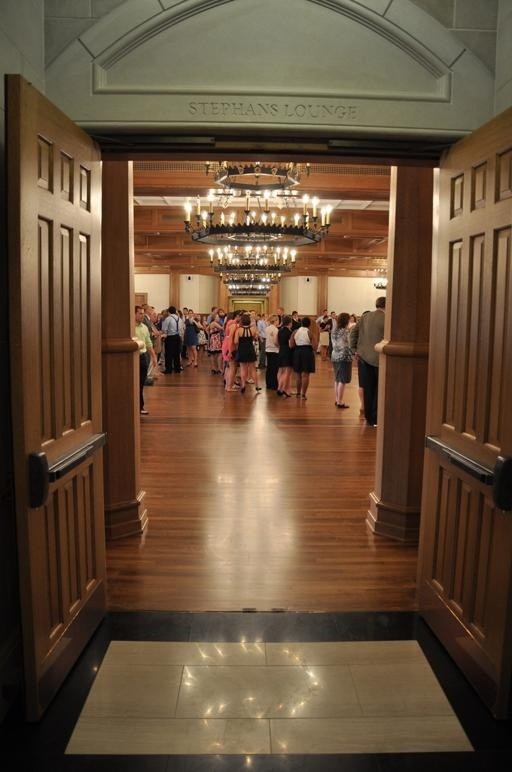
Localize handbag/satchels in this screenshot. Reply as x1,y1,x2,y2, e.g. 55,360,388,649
195,320,200,333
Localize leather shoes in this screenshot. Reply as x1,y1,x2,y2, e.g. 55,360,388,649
141,410,148,414
160,370,170,373
335,401,348,408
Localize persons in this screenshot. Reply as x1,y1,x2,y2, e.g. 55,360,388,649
347,295,385,428
131,292,371,418
133,304,159,416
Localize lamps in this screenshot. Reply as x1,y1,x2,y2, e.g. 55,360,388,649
183,160,334,295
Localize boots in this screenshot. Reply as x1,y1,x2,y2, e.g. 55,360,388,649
359,410,367,419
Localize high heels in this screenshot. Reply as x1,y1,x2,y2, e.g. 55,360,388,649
186,362,307,400
